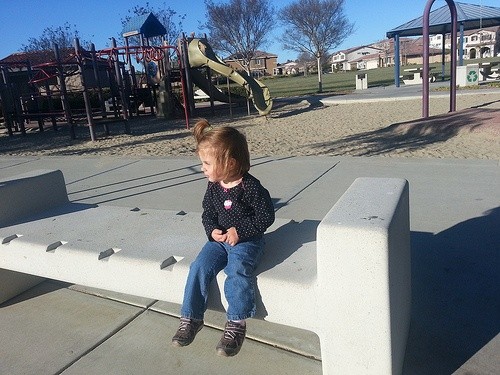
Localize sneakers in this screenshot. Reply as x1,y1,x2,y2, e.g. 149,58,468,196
215,320,246,358
172,317,204,347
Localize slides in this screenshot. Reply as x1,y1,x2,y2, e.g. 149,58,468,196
187,37,273,116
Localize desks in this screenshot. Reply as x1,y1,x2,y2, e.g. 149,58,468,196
403,67,437,79
468,62,500,80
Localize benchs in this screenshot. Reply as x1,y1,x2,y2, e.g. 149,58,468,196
491,69,499,78
0,169,411,375
399,72,442,84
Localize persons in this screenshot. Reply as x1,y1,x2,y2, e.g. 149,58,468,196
170,118,275,358
